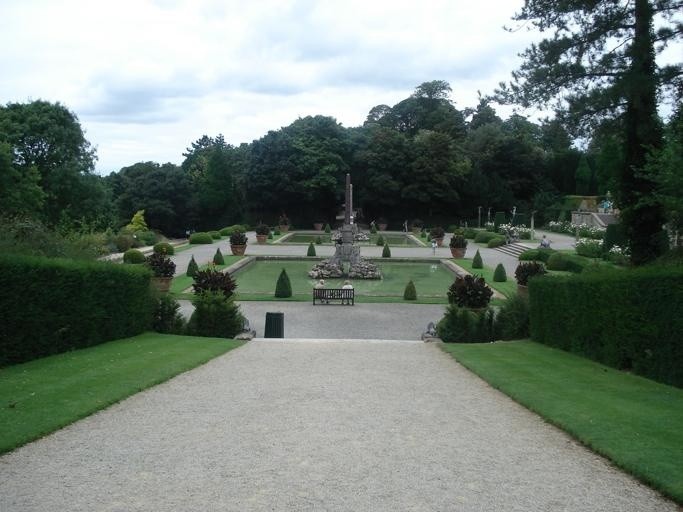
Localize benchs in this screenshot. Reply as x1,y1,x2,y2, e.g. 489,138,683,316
313,288,354,305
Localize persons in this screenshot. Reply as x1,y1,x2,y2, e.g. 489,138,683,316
316,279,330,303
604,199,617,214
541,235,547,248
431,238,438,255
341,280,353,305
186,226,196,239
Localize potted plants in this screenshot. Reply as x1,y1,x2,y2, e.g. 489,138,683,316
311,217,326,231
412,218,424,233
514,261,549,296
430,225,446,246
256,224,269,243
377,216,387,231
449,235,468,259
229,231,248,256
145,254,176,292
279,218,288,232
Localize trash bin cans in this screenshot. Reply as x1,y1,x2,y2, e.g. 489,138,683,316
264,312,284,338
